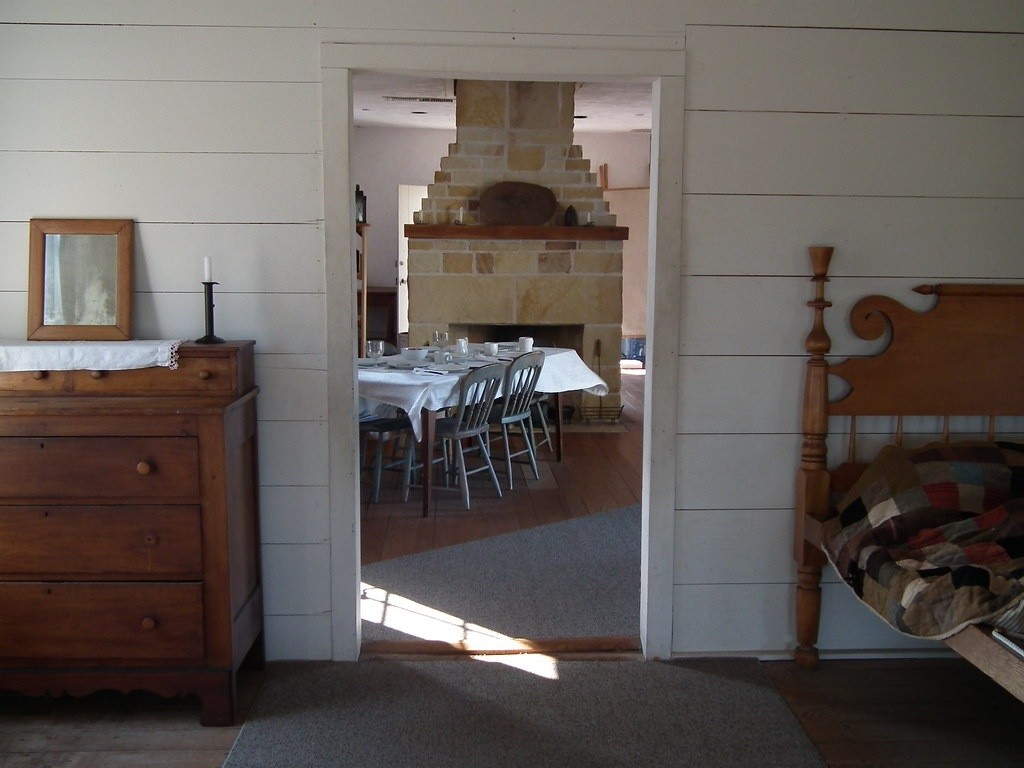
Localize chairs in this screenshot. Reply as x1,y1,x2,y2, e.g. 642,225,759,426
359,347,560,513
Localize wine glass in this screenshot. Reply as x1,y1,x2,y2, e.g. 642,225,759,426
433,331,448,351
365,340,384,369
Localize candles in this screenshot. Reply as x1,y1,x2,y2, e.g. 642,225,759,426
203,256,212,282
418,209,424,223
587,210,592,222
460,206,464,223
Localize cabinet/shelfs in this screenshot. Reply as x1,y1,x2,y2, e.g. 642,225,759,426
0,338,268,729
356,224,370,360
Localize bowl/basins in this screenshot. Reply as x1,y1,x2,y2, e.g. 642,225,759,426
401,347,428,361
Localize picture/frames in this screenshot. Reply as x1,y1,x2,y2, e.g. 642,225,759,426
26,217,137,341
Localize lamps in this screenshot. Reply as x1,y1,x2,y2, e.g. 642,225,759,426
355,183,367,225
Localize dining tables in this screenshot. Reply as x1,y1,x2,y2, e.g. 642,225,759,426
358,337,612,521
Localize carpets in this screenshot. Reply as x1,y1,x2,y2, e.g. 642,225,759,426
359,501,644,643
221,655,831,768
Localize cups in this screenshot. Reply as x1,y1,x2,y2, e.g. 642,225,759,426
519,337,533,352
434,351,452,364
454,339,468,358
484,342,498,355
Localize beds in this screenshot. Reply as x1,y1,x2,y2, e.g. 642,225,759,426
790,243,1024,703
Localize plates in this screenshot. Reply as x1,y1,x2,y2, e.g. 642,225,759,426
429,364,465,371
387,362,426,369
